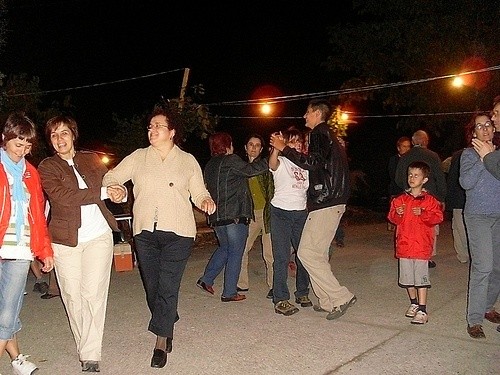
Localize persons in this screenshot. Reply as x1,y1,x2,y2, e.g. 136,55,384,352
386,161,444,325
30,258,48,293
196,131,270,301
331,216,345,249
268,127,313,317
458,111,500,338
101,108,216,368
23,290,28,296
395,131,445,268
0,110,38,375
446,122,473,264
472,97,500,333
41,193,60,300
35,116,127,371
286,129,312,278
390,136,411,196
239,133,275,298
272,99,357,320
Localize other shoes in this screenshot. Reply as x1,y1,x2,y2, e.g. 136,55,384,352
41,293,57,300
220,293,246,303
267,289,274,299
466,323,485,339
428,261,436,268
33,282,50,294
236,287,249,291
313,304,325,311
196,280,215,295
327,295,358,320
484,310,500,324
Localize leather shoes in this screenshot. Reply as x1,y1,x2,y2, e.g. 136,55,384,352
167,337,173,352
151,348,167,368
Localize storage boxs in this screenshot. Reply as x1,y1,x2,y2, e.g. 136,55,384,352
114,243,133,271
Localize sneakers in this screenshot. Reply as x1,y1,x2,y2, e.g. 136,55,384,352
405,304,419,317
274,300,299,315
295,295,313,307
411,312,429,324
9,354,38,375
81,359,99,372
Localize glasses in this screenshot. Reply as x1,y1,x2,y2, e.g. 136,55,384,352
147,124,169,130
476,121,493,130
